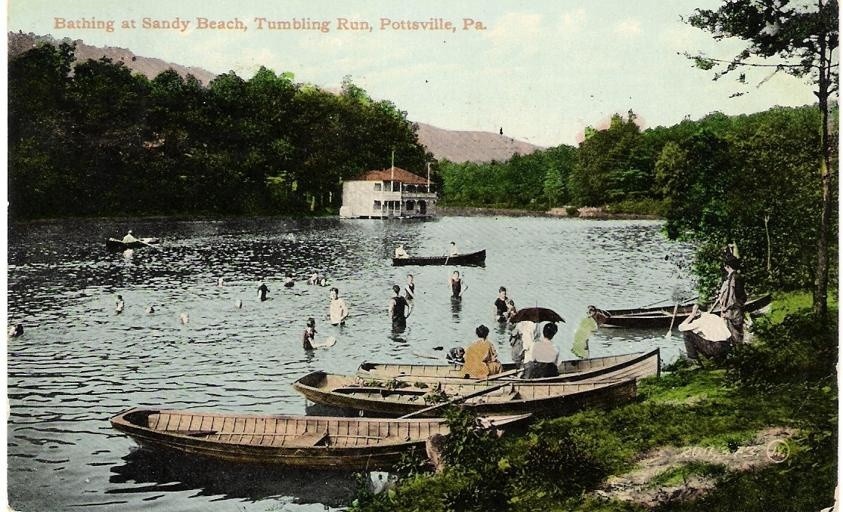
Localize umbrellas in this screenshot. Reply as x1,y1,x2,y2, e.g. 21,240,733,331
509,302,566,326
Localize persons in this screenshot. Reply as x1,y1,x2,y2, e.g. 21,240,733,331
679,303,732,371
719,256,747,356
511,321,541,357
460,324,505,376
122,230,139,243
495,286,508,323
304,242,470,353
524,322,559,367
503,298,518,326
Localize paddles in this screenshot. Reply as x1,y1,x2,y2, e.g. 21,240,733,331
665,301,680,339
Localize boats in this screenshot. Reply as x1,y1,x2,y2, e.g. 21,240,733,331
107,238,161,248
590,293,771,329
391,249,486,265
110,406,531,473
